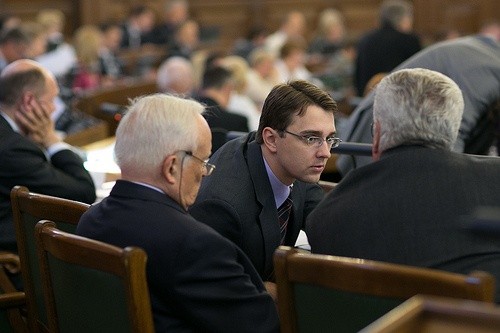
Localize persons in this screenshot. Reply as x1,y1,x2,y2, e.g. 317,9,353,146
0,0,500,307
75,94,281,333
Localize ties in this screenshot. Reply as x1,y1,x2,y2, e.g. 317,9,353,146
277,186,293,246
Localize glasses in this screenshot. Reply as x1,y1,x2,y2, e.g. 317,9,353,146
163,149,216,177
279,129,342,148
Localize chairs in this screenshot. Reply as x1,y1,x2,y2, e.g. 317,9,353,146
0,186,500,333
